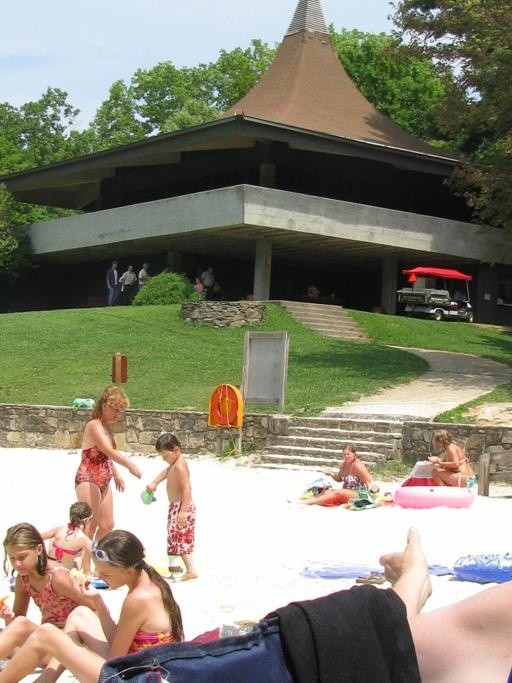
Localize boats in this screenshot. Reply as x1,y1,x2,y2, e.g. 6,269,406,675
393,460,476,509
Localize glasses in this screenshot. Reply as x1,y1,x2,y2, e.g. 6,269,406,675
90,540,122,568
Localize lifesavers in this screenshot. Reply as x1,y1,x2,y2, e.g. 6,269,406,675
395,485,475,508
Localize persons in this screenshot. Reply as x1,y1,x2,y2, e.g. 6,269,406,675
201,264,216,300
138,262,152,290
98,526,510,683
106,259,121,306
146,430,198,582
286,442,372,506
74,380,144,575
193,277,204,292
427,428,475,488
119,264,137,306
0,523,99,670
0,528,184,682
39,501,93,576
